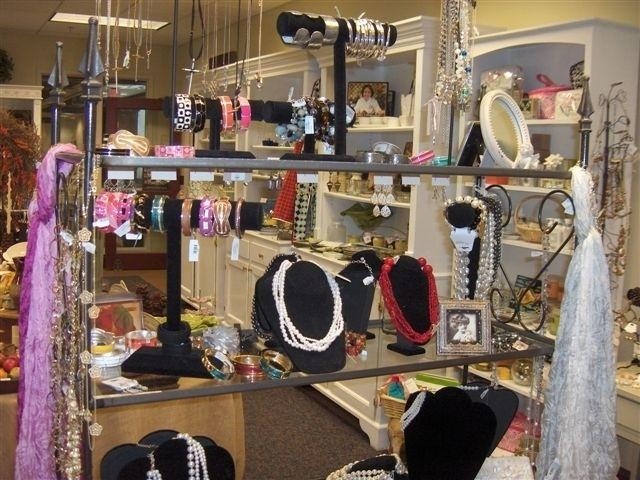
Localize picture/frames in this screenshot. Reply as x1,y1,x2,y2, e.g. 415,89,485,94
347,81,389,117
436,299,492,357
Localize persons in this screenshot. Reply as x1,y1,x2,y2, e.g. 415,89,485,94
353,83,387,118
329,249,382,338
451,312,477,343
252,254,347,373
399,385,497,479
101,428,216,480
250,251,298,341
114,431,237,480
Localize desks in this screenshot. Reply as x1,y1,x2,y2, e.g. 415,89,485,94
90,329,247,479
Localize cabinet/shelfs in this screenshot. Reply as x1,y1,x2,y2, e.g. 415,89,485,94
452,18,640,480
170,14,457,450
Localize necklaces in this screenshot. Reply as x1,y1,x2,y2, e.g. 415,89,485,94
274,258,345,353
434,0,477,117
144,433,210,480
401,391,428,430
92,1,266,99
322,452,409,480
442,192,505,301
251,257,273,342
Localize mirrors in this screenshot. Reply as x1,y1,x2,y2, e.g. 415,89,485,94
480,89,532,168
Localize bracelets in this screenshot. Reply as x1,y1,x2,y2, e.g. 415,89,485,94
231,353,266,377
92,86,337,240
258,349,294,381
203,347,235,380
276,6,397,63
